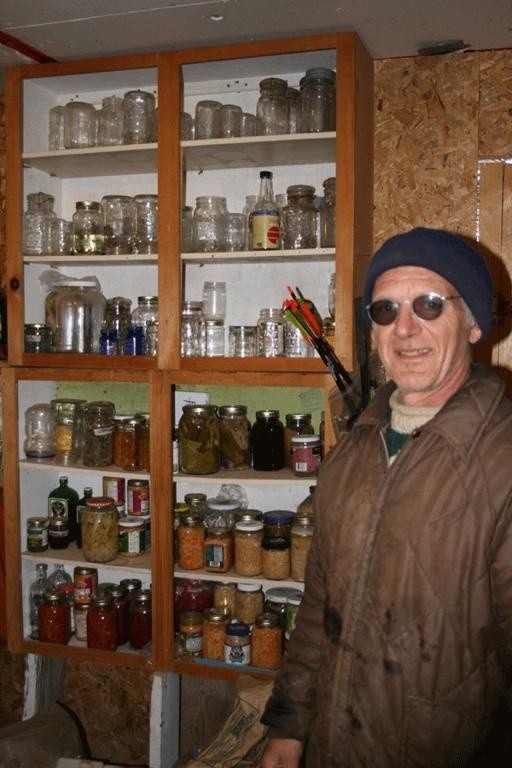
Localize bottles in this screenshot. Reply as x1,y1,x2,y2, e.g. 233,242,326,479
182,171,336,252
75,487,93,549
181,273,336,358
24,192,158,254
24,280,158,356
28,563,55,640
48,563,72,589
181,67,336,141
48,476,79,541
49,90,158,150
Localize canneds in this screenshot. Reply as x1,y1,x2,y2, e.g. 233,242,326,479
28,561,150,653
23,274,158,357
23,190,158,256
182,177,338,250
49,398,150,476
258,67,337,135
178,271,338,359
176,401,326,478
169,577,306,672
24,473,152,563
173,481,323,584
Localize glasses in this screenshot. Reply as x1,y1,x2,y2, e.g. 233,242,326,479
365,290,460,326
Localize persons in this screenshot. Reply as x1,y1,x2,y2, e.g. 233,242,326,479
258,226,512,768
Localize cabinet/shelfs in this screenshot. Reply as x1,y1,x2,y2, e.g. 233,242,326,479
2,29,375,372
0,367,372,682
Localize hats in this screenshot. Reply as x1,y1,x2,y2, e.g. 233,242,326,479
365,226,492,340
